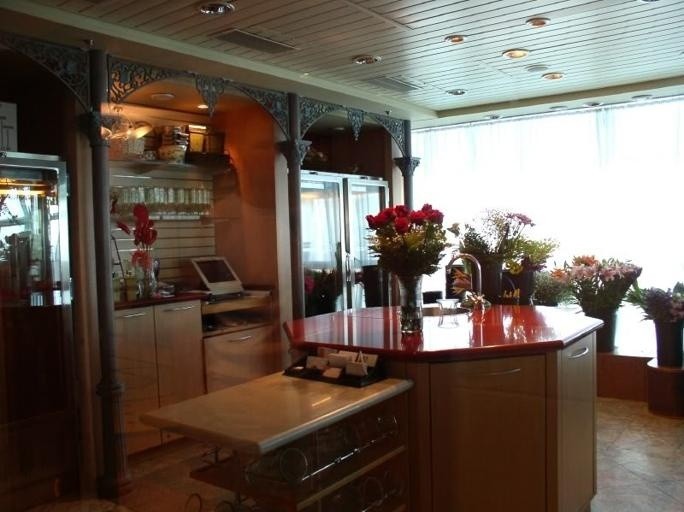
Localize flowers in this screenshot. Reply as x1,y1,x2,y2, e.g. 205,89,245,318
550,255,642,312
362,204,460,276
625,279,683,324
446,207,570,305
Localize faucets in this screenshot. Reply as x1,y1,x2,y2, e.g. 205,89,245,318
446,254,484,297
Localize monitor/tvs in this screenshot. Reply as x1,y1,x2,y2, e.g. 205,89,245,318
189,257,242,289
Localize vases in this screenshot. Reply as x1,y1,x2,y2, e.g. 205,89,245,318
586,313,616,352
652,319,683,367
394,273,424,334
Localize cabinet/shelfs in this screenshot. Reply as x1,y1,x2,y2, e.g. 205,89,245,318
202,299,281,390
141,368,415,512
105,145,237,224
282,303,604,512
114,298,202,453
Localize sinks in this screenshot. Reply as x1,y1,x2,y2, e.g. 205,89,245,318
422,307,470,317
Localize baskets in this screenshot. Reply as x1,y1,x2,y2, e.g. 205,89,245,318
109,138,145,160
